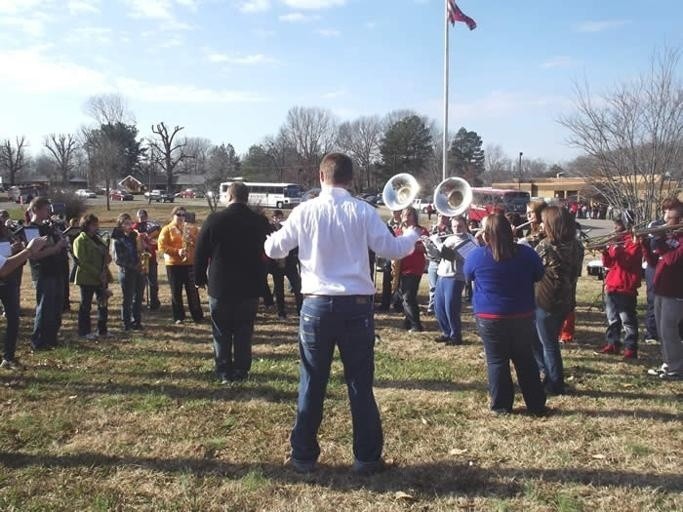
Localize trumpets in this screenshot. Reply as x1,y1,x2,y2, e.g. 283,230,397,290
579,231,615,255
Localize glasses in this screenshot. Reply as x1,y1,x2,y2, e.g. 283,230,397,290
175,213,186,217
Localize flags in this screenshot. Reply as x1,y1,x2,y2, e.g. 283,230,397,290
446,0,477,30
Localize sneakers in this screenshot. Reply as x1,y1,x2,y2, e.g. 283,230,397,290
175,319,182,324
445,339,463,346
283,457,317,483
596,343,620,355
407,327,423,333
80,333,97,340
659,371,683,379
352,456,395,476
648,363,670,377
434,333,450,342
96,330,114,337
123,320,147,332
614,347,637,362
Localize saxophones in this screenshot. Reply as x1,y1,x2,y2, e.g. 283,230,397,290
388,220,409,294
96,231,115,308
131,228,151,275
180,221,193,262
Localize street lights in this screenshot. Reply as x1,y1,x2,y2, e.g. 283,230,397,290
518,151,524,190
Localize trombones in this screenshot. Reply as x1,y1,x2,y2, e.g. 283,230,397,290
582,223,683,250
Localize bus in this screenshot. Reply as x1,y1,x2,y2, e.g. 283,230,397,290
217,181,304,210
465,188,532,230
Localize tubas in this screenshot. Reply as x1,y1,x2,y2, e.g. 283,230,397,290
383,173,419,219
432,177,474,218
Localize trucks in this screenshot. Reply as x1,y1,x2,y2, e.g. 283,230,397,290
6,184,44,203
410,198,435,214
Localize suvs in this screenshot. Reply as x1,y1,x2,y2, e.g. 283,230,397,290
143,187,176,204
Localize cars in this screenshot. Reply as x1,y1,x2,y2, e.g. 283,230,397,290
179,188,205,199
354,191,383,205
75,188,97,199
109,191,134,201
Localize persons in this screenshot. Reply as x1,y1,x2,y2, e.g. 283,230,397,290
262,153,422,472
6,180,683,416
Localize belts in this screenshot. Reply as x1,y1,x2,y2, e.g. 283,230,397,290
301,292,374,303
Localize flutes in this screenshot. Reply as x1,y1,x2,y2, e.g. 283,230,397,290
417,233,463,242
514,219,538,231
